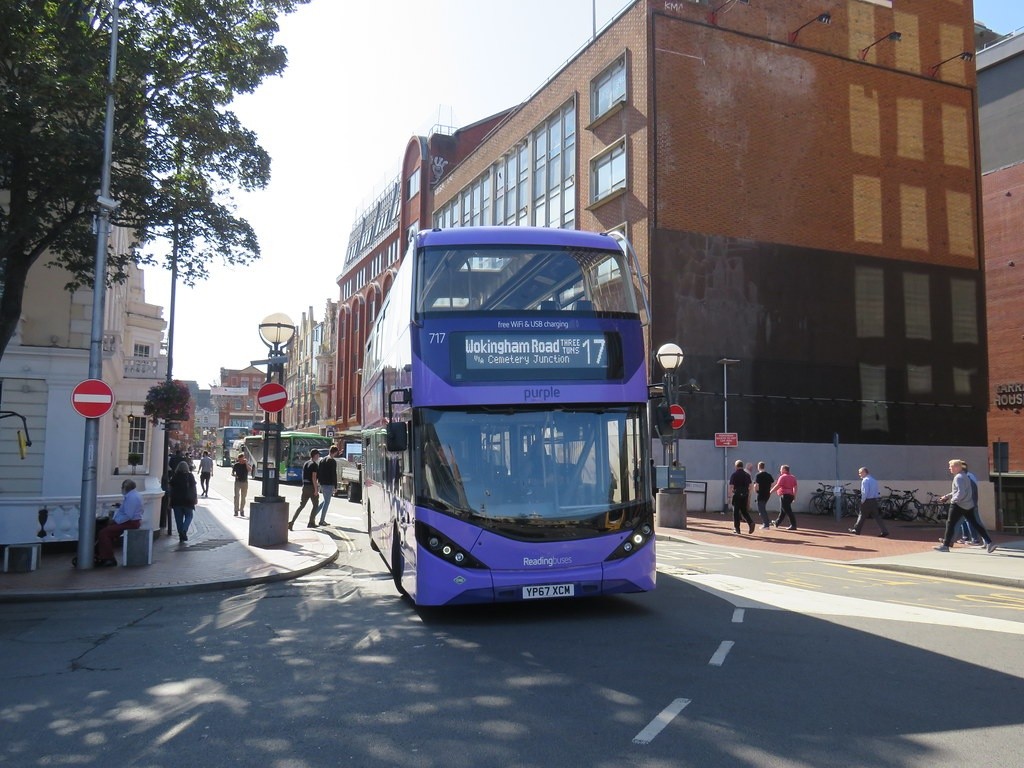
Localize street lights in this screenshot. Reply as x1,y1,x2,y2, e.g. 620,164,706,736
253,312,296,503
717,358,740,514
655,342,685,494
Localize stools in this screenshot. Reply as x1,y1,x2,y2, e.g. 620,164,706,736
3,544,41,572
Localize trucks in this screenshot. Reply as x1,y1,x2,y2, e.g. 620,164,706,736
316,457,362,503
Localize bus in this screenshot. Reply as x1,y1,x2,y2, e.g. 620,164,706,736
214,426,250,468
243,432,334,483
359,225,672,607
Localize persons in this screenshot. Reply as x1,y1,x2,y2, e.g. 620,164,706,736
650,458,659,500
729,459,756,534
754,462,774,530
669,459,685,489
847,467,889,537
288,445,339,531
169,450,193,474
770,464,799,530
197,450,213,497
609,461,617,504
740,463,753,522
169,461,198,543
231,453,252,517
933,459,999,553
98,479,144,566
435,442,474,503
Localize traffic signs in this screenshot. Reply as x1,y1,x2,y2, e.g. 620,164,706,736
715,433,739,448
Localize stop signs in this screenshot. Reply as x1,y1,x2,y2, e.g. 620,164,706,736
68,379,115,420
668,404,686,430
258,381,289,414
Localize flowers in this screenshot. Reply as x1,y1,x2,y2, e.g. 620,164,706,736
141,375,193,428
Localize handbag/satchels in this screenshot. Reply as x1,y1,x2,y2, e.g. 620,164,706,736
186,474,197,504
611,478,617,489
112,509,130,524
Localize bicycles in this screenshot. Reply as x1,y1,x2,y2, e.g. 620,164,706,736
807,480,950,526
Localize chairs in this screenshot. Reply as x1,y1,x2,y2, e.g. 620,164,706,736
572,300,596,311
541,300,562,311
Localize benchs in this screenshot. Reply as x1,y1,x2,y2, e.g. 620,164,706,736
120,527,152,567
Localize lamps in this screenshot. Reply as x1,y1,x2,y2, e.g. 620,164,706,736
931,52,975,75
790,14,830,43
705,0,749,22
127,402,135,423
859,33,901,61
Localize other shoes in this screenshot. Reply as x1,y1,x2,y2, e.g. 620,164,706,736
965,540,979,546
848,527,860,535
987,543,998,553
981,542,987,548
319,522,330,526
771,519,779,527
957,537,969,543
234,511,238,516
308,523,318,527
99,561,117,566
749,522,755,534
734,528,740,533
759,526,769,530
933,546,949,552
289,522,293,531
786,526,796,530
240,511,244,517
179,530,188,543
938,538,953,546
878,533,889,538
